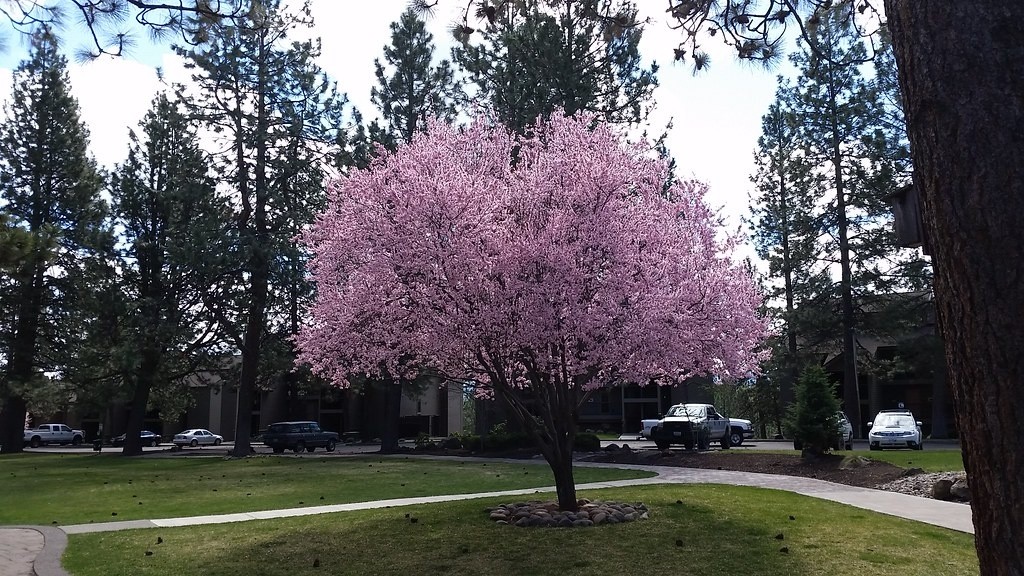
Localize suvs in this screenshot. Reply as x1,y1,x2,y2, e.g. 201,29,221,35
264,421,340,453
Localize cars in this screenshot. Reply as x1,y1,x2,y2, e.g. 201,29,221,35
793,411,854,451
111,430,162,448
867,409,923,450
650,404,733,451
172,428,224,447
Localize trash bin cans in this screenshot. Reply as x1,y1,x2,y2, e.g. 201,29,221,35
93,439,100,451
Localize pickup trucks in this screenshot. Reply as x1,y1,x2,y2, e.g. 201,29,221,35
24,424,86,448
639,411,754,447
